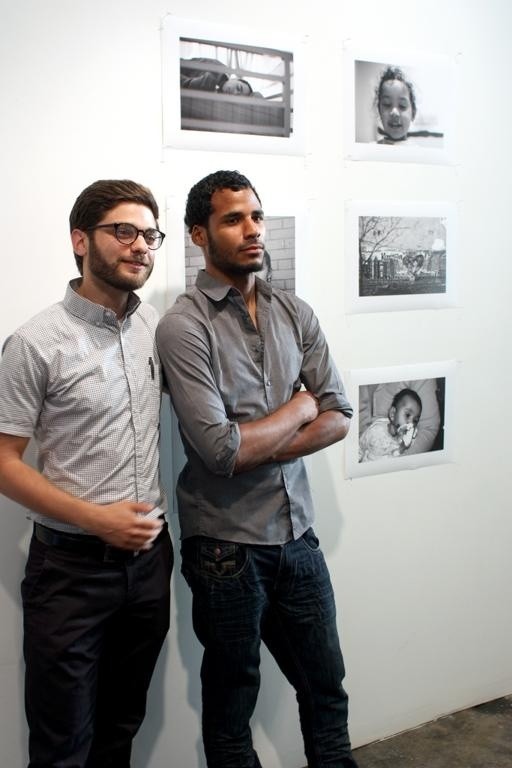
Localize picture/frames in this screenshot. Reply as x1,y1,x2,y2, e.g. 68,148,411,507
343,38,471,164
161,12,310,153
159,393,187,513
344,197,466,313
166,198,309,316
341,362,461,478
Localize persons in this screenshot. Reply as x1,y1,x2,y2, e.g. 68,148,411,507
0,179,174,767
358,388,424,463
253,248,274,285
180,57,253,96
155,170,354,767
374,67,417,144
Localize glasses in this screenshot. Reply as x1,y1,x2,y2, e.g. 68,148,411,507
86,223,165,250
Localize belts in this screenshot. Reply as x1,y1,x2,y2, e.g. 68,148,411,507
36,512,165,563
